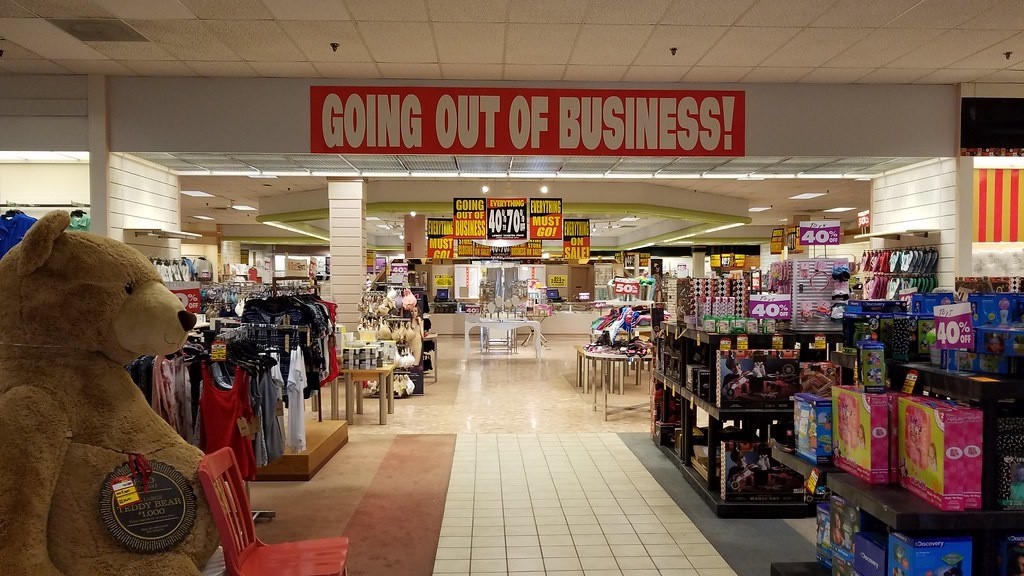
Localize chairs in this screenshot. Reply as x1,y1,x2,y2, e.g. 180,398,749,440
197,447,349,576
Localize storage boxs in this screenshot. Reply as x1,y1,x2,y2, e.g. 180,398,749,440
717,349,808,504
795,291,1024,575
687,363,709,399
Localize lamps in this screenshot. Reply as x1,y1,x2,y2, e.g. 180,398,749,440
539,182,548,193
608,222,612,229
482,182,489,193
592,225,597,233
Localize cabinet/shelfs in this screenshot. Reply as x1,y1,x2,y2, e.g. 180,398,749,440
676,277,750,328
854,227,940,280
650,320,844,520
588,300,667,371
772,257,848,331
122,228,202,261
606,283,654,344
770,275,1024,576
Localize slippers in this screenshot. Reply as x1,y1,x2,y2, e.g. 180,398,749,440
860,251,938,274
862,275,938,301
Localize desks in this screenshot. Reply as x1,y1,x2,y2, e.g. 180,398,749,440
462,314,542,363
339,339,396,369
520,313,547,347
340,361,397,425
574,344,652,421
423,330,438,383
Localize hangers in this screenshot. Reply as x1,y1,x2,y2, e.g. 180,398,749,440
70,205,86,220
0,205,24,218
183,284,338,375
150,256,208,269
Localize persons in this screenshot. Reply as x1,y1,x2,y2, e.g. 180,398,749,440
721,356,754,398
403,305,425,367
757,454,771,471
726,446,757,497
751,361,766,378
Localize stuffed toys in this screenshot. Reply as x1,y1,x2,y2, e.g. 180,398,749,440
0,209,227,576
